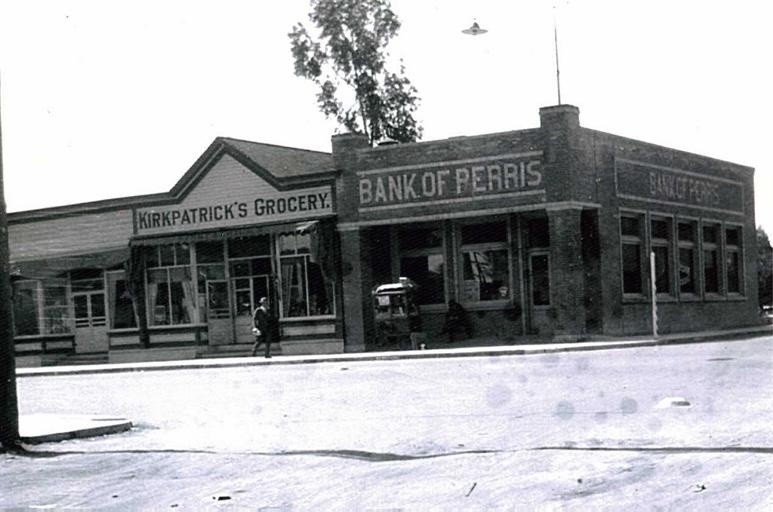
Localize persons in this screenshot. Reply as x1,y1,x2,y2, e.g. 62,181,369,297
251,297,276,359
440,299,474,340
